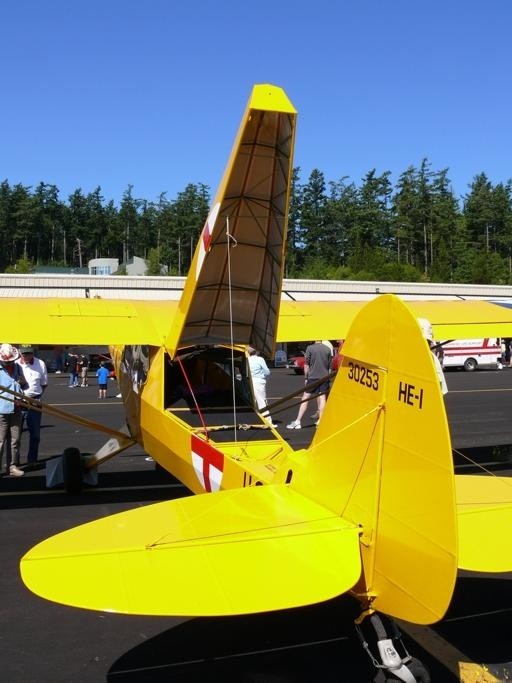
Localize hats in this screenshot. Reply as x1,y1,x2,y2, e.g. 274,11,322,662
417,318,434,343
0,343,20,362
19,344,34,354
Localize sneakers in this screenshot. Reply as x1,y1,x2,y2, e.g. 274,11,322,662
285,419,302,430
5,465,25,477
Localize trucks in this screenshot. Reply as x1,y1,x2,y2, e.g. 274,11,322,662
429,337,507,372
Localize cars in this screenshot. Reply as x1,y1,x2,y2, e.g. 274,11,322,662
284,346,339,376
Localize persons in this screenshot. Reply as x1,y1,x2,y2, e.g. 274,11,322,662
0,344,30,476
66,351,88,388
416,318,448,396
95,361,110,398
247,347,278,429
13,344,48,471
434,340,443,367
286,341,334,430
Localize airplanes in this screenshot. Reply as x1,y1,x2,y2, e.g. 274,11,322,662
0,83,512,681
